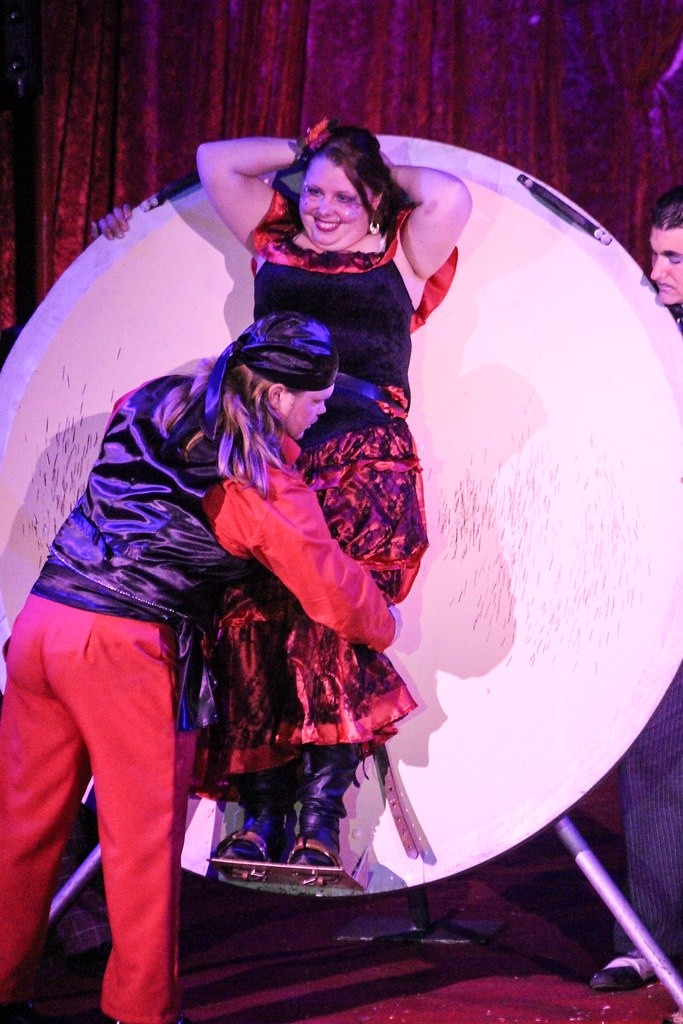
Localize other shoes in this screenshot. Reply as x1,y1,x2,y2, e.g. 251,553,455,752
0,1001,47,1024
590,954,656,990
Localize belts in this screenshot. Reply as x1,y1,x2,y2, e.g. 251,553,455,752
335,373,391,401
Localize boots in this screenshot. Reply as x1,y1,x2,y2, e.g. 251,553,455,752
220,767,286,862
291,742,369,869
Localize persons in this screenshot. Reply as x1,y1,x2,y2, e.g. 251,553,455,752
0,313,402,1024
587,185,683,987
176,116,471,885
